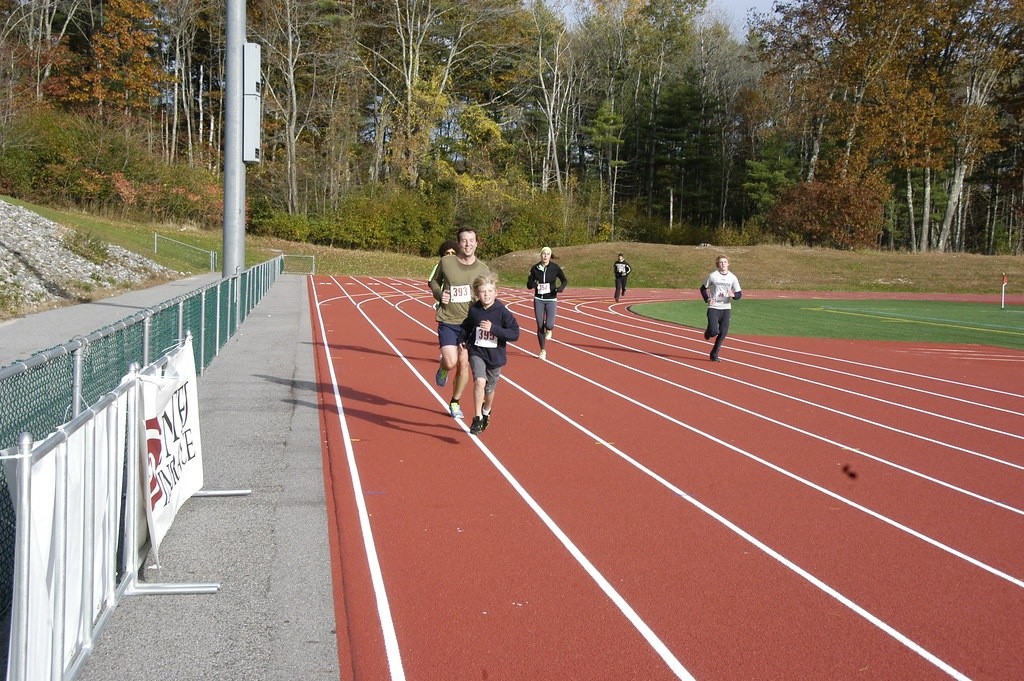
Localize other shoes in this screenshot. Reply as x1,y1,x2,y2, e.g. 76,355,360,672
622,290,625,296
710,356,721,362
615,297,619,302
704,331,710,340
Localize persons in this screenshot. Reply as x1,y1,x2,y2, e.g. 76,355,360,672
527,247,567,360
456,273,520,436
428,241,459,361
700,255,742,362
429,227,490,418
613,253,632,302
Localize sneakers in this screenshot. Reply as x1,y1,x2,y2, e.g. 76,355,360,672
545,329,553,341
470,416,484,436
436,367,449,386
540,350,546,359
481,399,492,429
449,402,464,419
